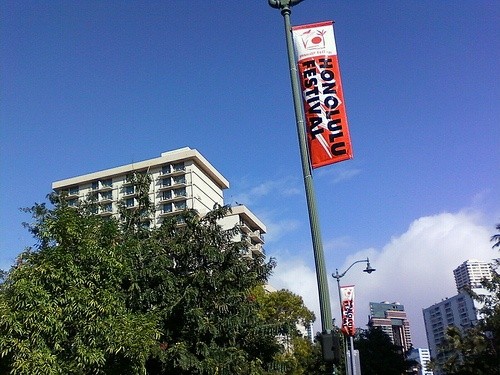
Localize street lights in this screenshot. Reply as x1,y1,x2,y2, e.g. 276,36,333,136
267,0,341,375
332,257,375,374
